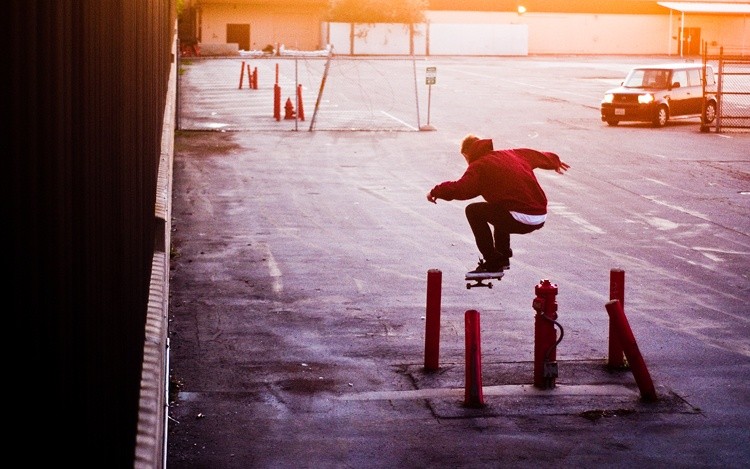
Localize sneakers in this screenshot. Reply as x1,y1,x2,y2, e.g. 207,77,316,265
465,262,510,277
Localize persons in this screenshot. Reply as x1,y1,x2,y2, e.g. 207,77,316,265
426,134,571,279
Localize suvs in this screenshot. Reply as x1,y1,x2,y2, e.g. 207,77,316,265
601,63,719,128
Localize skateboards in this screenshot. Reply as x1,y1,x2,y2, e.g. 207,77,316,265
462,262,511,288
